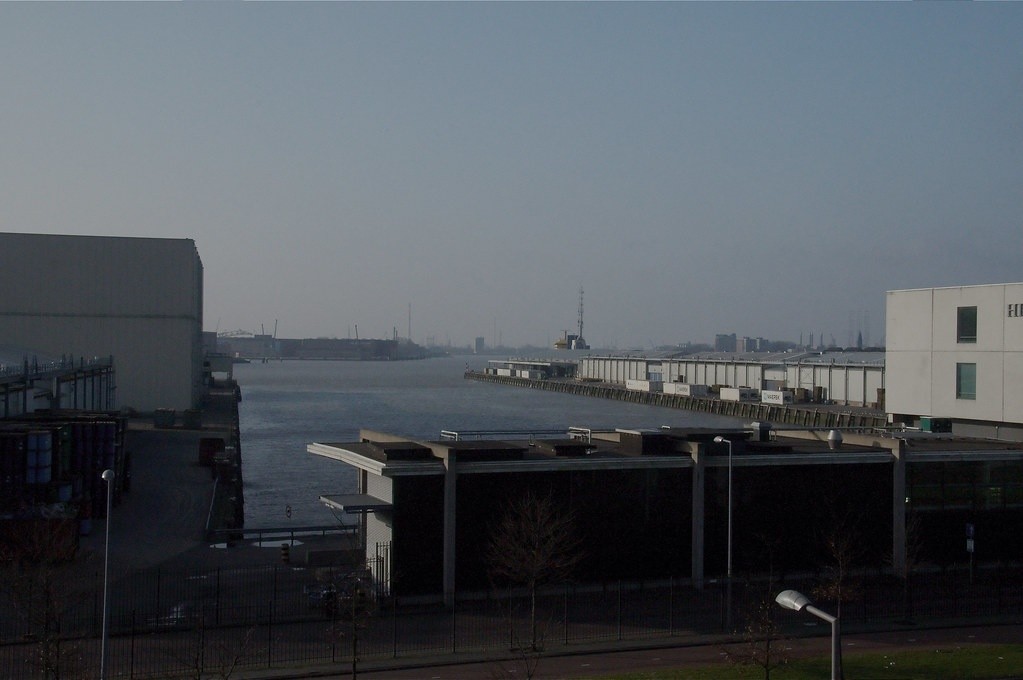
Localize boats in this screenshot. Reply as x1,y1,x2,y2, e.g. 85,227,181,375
233,357,251,363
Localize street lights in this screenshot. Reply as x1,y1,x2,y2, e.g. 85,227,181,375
99,469,116,680
775,590,845,679
713,435,734,631
